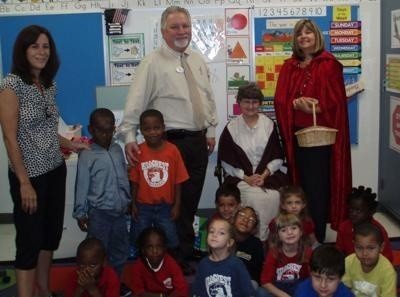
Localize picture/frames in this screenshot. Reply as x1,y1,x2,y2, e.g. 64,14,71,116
108,33,145,61
109,61,140,86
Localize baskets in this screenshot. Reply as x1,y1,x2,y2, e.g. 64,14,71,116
295,101,339,147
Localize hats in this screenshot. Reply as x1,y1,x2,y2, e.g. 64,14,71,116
236,83,264,102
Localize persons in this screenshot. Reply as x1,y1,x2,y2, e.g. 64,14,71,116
293,246,356,297
259,213,313,297
230,207,264,291
0,25,89,297
341,223,398,297
274,20,352,246
208,182,241,229
217,85,285,243
190,218,257,297
128,109,190,260
118,6,219,277
335,186,393,263
71,108,133,297
130,227,188,297
65,238,121,297
267,186,319,248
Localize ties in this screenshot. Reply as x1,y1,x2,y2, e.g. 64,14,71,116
180,52,206,131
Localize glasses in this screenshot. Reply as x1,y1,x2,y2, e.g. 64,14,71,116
238,211,257,223
